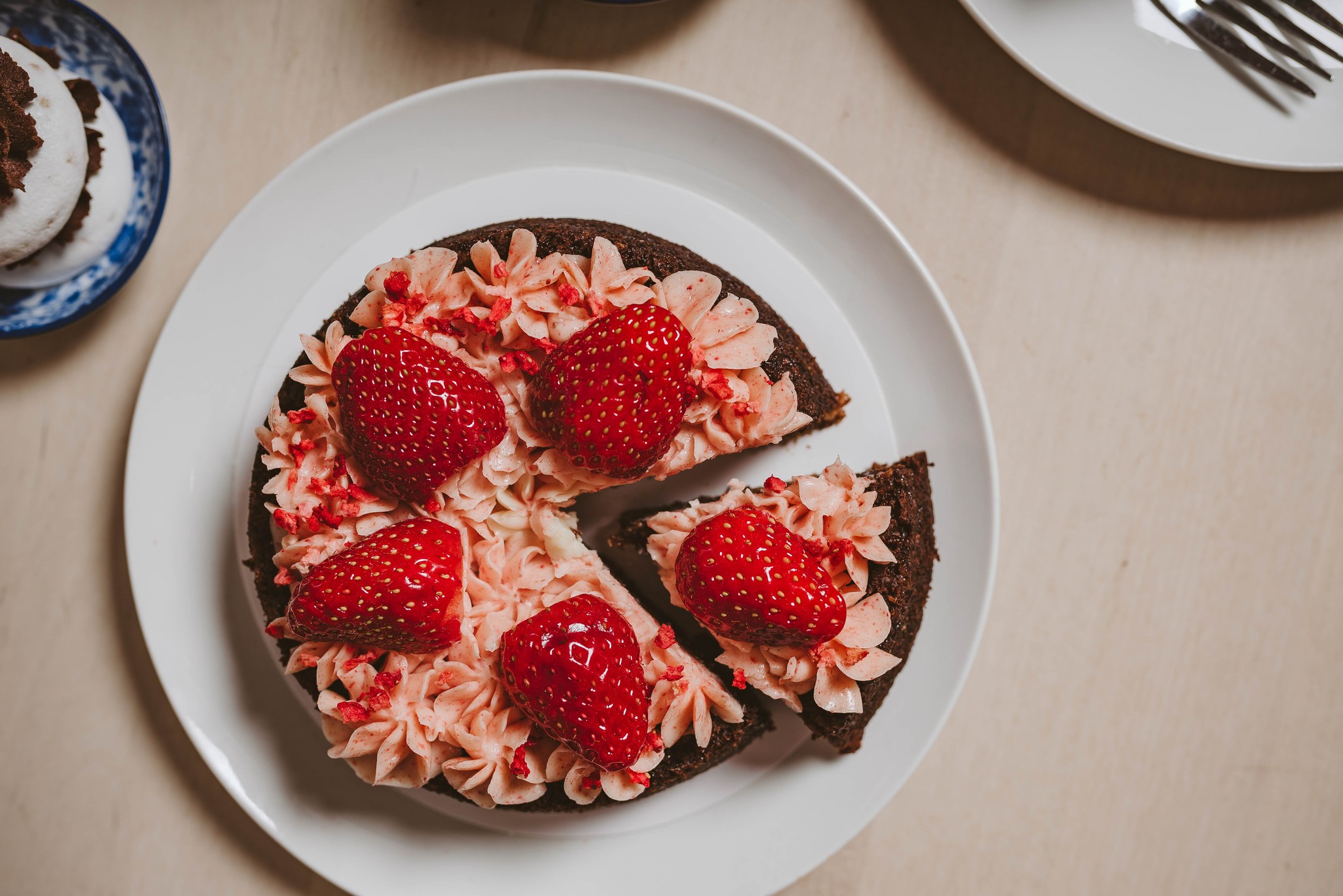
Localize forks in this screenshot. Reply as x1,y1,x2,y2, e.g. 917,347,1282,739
1157,0,1343,98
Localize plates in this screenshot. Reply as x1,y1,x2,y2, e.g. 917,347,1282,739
0,0,171,341
122,69,1001,896
956,0,1343,171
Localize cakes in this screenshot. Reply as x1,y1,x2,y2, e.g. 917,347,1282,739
244,217,938,811
0,24,133,281
